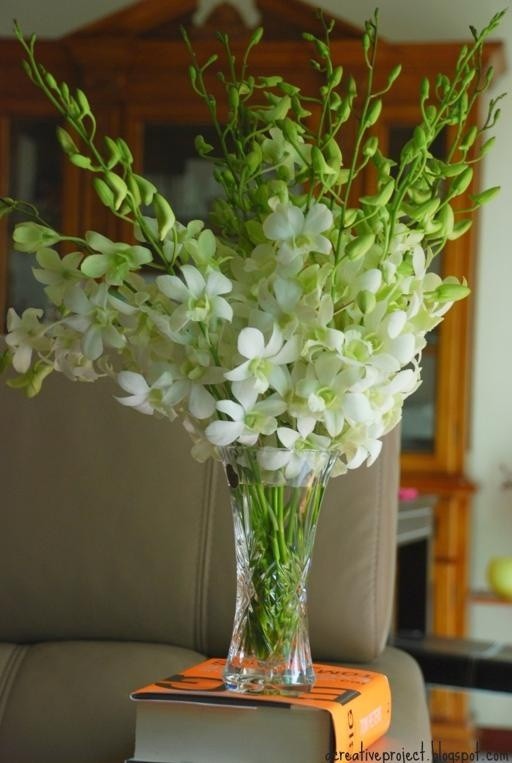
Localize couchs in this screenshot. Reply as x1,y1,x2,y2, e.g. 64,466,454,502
2,360,435,763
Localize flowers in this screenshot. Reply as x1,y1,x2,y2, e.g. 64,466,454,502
0,3,510,486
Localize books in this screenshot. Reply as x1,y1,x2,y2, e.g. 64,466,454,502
128,657,394,762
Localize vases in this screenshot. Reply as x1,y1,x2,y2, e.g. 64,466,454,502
214,446,340,693
